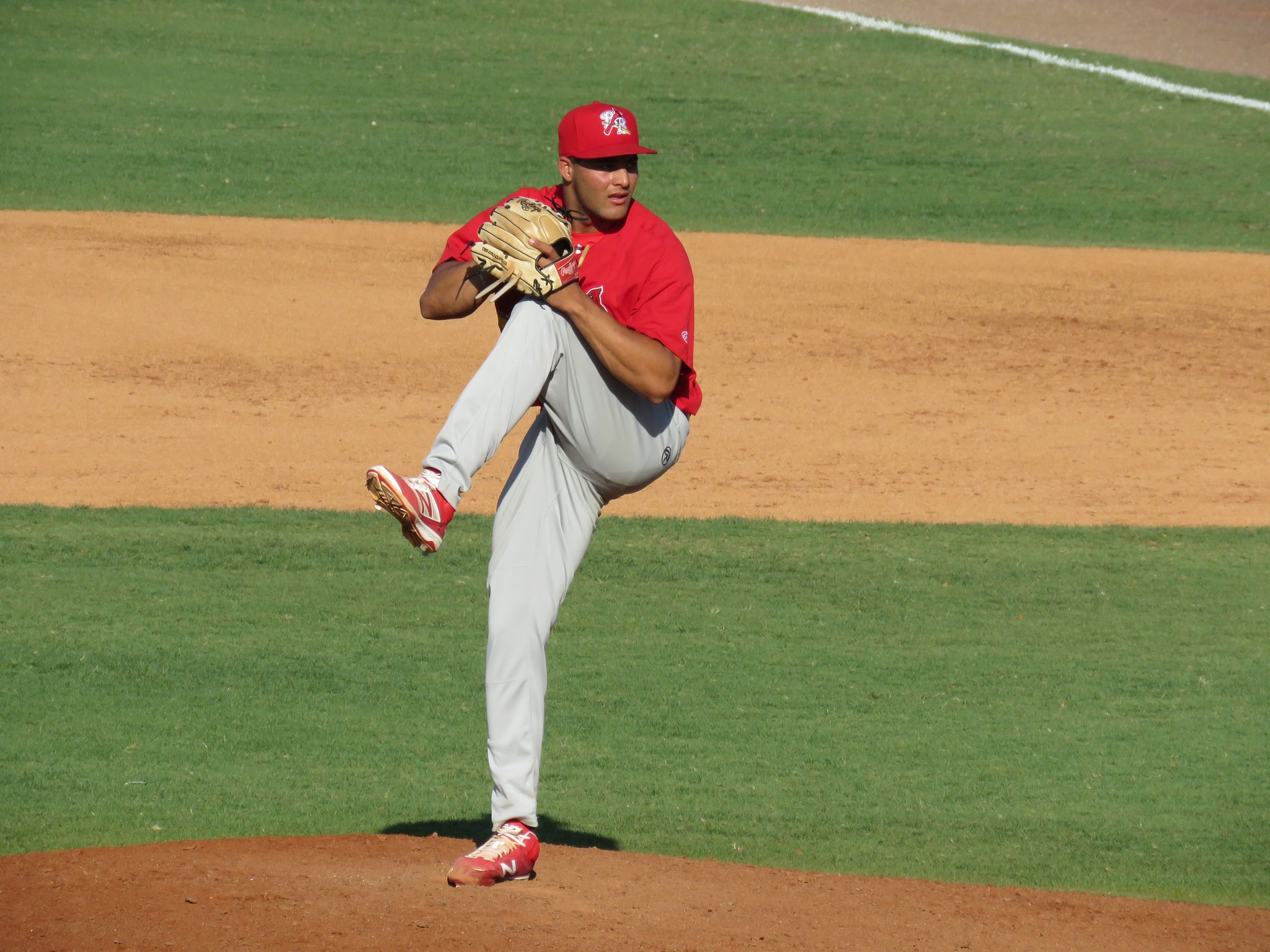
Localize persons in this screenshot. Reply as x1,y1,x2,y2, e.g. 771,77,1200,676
363,103,703,886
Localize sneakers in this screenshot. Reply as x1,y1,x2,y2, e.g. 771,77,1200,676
365,465,455,555
448,816,540,886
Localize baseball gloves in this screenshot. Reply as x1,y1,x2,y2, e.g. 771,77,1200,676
471,195,579,297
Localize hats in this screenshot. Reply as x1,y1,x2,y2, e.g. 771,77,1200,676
558,101,658,159
